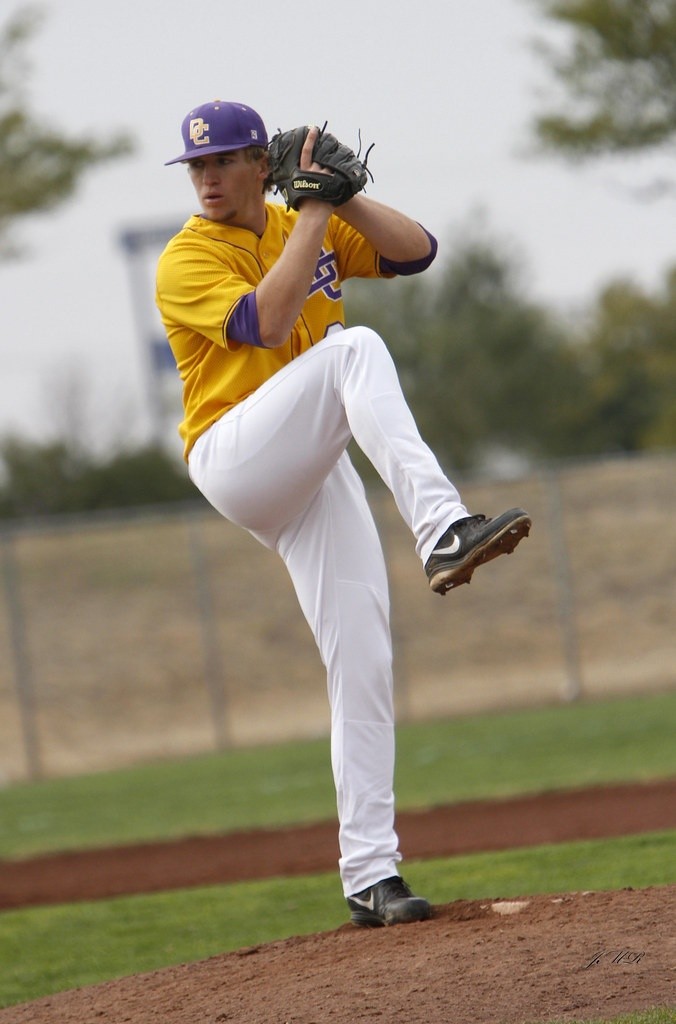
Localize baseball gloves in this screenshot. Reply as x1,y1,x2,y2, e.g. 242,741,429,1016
266,124,370,212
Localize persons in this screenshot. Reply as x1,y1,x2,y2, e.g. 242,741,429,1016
154,100,532,927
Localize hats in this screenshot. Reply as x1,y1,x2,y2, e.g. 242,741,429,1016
164,98,268,166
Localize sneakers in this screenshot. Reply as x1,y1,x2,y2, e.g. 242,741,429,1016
424,507,532,595
346,876,431,927
